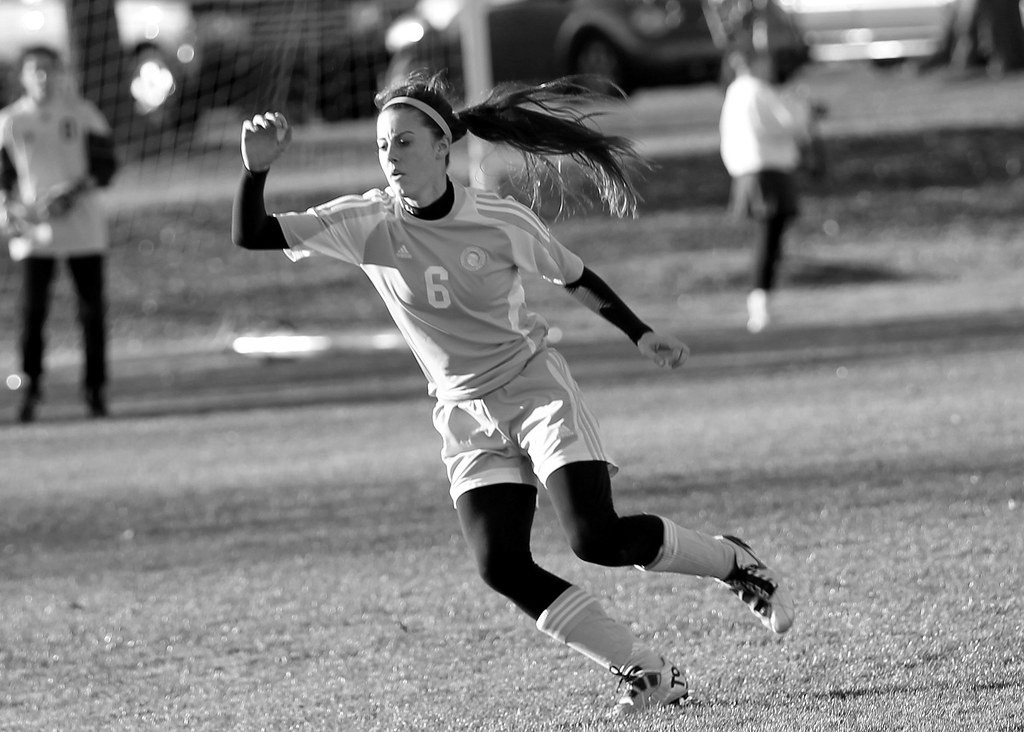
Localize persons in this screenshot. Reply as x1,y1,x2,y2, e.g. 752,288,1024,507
0,45,119,421
231,75,795,717
720,44,814,330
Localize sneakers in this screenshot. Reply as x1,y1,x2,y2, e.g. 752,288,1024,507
609,655,689,710
714,534,796,635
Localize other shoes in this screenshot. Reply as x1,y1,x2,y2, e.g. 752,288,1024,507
20,387,42,423
746,287,770,334
83,390,110,418
